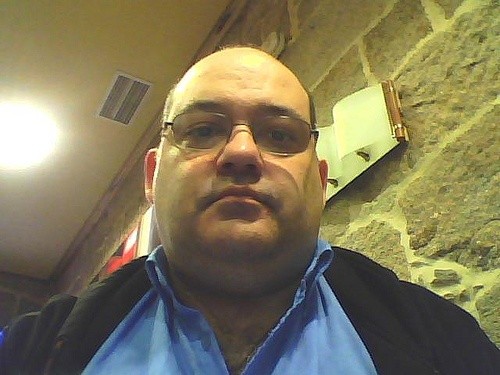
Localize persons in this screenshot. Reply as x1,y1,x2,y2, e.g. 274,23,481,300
0,46,500,375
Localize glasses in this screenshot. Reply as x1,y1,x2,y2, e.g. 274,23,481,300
160,109,320,154
